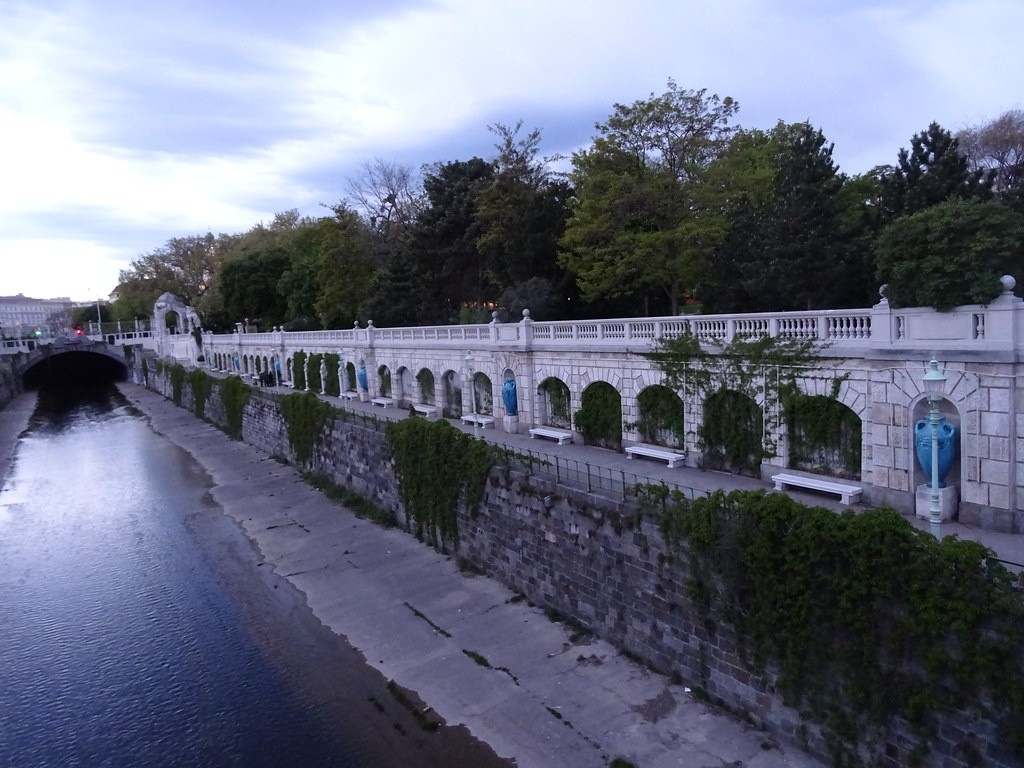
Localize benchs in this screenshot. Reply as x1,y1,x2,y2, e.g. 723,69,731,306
461,416,494,429
625,446,685,469
529,428,574,446
414,407,436,418
371,398,393,408
771,473,864,505
283,382,292,388
252,376,259,382
210,368,248,378
341,391,360,401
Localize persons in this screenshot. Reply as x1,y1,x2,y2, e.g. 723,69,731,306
302,358,311,391
259,370,276,387
319,359,328,394
289,356,295,388
337,360,344,399
